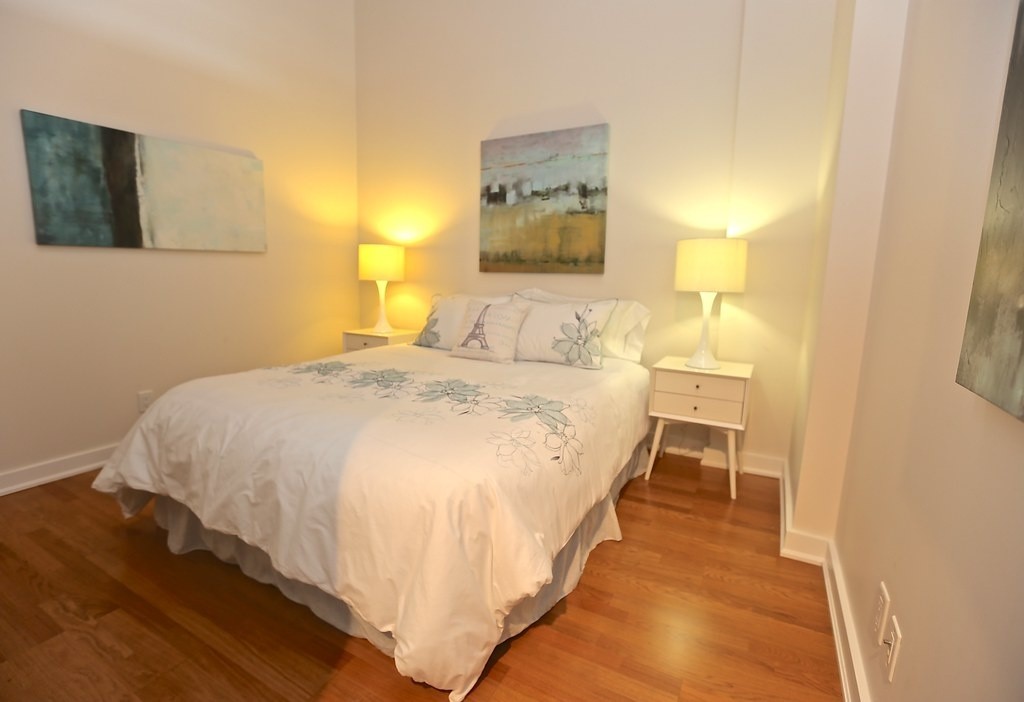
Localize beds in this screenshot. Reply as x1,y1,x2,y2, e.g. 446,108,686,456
89,342,651,702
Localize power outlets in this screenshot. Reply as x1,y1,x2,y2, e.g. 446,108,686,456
878,615,902,684
868,581,890,646
138,390,156,414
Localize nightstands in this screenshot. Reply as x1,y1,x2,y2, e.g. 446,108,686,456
643,355,755,502
342,328,421,356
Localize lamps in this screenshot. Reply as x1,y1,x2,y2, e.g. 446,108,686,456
674,238,749,369
358,244,406,333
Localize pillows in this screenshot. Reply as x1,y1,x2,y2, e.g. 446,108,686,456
509,292,619,369
448,298,529,366
412,292,512,350
